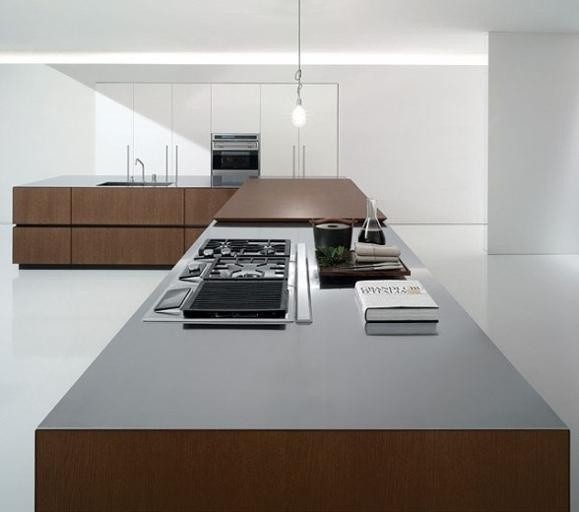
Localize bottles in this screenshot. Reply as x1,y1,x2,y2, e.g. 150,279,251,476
359,197,385,245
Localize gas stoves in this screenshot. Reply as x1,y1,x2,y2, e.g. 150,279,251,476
142,233,315,329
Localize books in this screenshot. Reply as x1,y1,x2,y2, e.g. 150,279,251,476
355,280,440,322
361,318,440,337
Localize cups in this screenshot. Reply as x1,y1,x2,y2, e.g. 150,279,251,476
314,220,353,253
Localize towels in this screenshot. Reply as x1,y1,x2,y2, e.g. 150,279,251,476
355,241,401,263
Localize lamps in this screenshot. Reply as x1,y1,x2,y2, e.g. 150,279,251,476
291,1,306,128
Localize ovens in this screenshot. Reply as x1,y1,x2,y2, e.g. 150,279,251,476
211,133,260,178
